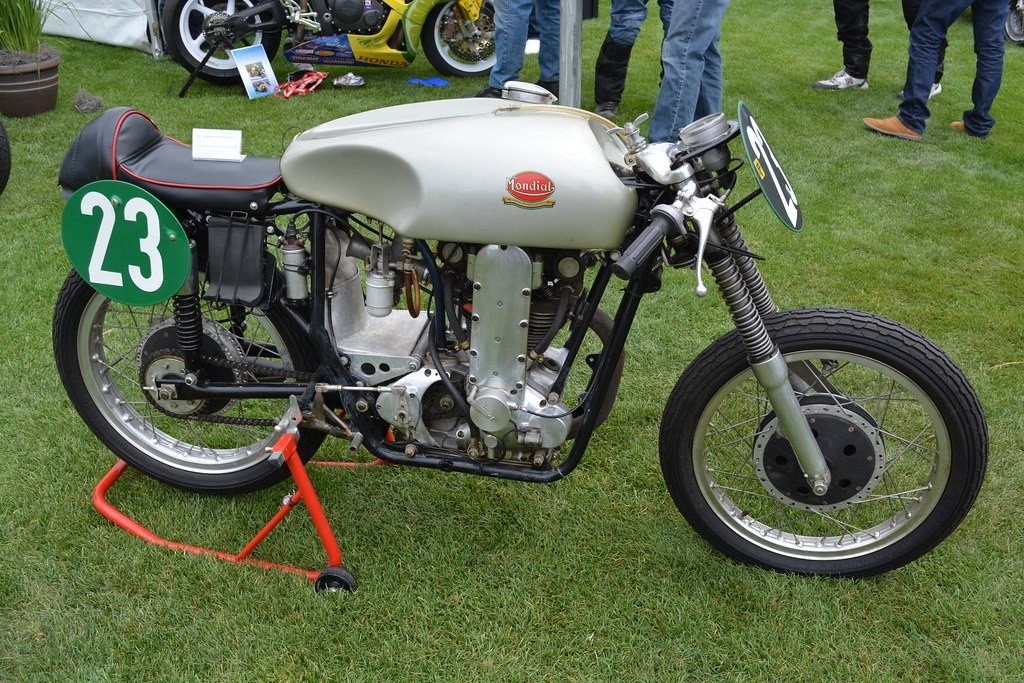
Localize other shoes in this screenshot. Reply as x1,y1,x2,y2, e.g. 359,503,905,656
594,102,619,119
535,80,560,99
950,121,965,129
476,86,507,98
865,115,922,141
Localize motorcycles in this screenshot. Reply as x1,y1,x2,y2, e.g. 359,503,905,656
47,100,991,585
161,0,497,99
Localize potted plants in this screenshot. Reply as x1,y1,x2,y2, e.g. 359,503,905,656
0,0,97,118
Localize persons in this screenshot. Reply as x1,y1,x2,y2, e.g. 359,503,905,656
473,0,560,102
863,0,1009,141
648,0,730,143
813,0,949,99
593,0,676,120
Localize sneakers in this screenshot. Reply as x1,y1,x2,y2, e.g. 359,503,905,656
897,84,942,99
813,72,868,91
